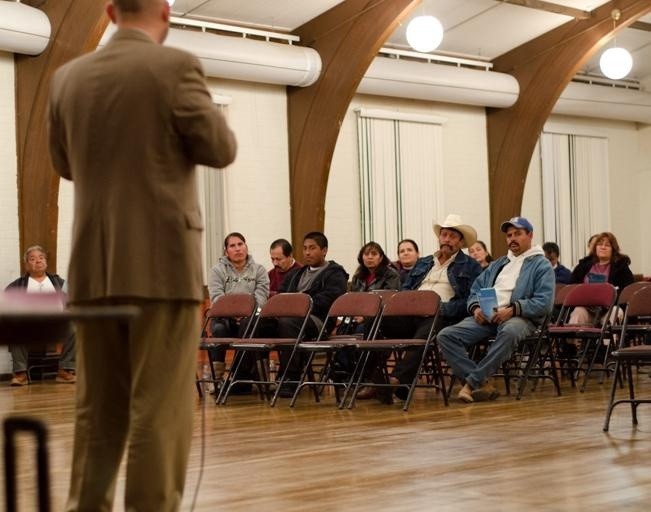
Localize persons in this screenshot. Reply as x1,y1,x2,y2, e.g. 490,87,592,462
5,246,77,386
48,0,236,512
209,216,555,402
542,232,635,376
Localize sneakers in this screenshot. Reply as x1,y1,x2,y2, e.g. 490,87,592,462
458,387,474,403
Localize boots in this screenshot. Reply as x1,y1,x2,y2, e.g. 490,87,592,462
210,361,225,394
257,358,279,393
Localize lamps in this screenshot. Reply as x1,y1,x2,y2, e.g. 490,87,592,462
600,9,632,79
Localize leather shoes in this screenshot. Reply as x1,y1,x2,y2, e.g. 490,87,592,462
11,370,29,385
56,367,78,383
357,386,376,399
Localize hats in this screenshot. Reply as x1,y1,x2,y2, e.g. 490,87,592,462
434,214,478,248
500,216,534,233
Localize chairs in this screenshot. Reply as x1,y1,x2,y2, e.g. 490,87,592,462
289,292,390,410
220,291,321,407
603,284,650,432
195,292,267,406
347,289,450,411
451,275,649,401
